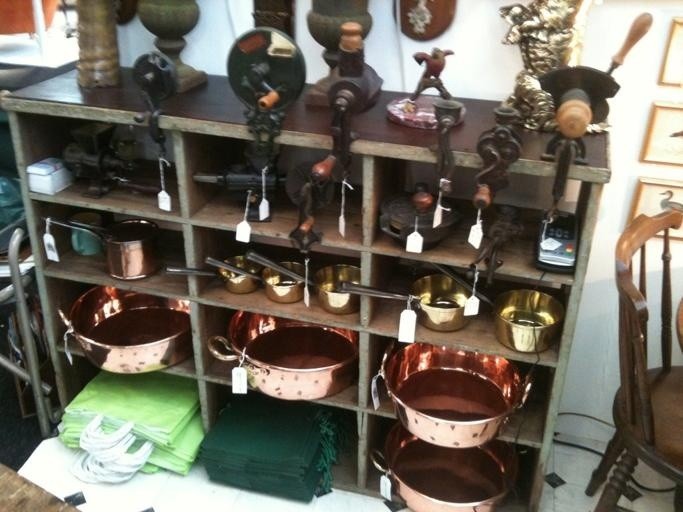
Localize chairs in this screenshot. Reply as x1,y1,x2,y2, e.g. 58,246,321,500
585,208,683,512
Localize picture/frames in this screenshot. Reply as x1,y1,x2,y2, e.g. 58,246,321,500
655,18,683,87
637,102,683,167
627,176,683,241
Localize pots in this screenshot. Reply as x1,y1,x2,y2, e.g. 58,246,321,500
57,284,193,375
380,338,537,449
166,254,264,294
205,309,358,401
337,273,475,332
431,262,566,353
203,255,306,303
42,215,167,281
246,249,361,315
369,421,529,512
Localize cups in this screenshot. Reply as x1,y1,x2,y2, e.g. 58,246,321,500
71,213,104,257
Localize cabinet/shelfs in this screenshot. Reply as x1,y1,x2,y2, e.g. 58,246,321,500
0,66,612,512
0,216,61,438
